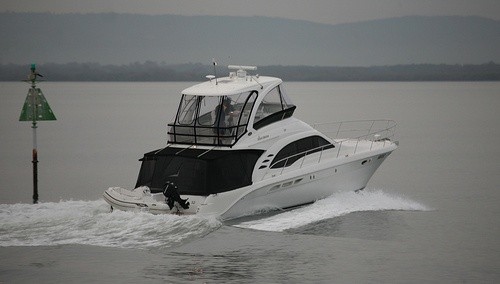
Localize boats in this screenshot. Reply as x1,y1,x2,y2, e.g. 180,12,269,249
102,59,400,224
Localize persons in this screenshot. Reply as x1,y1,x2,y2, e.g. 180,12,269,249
212,95,238,138
